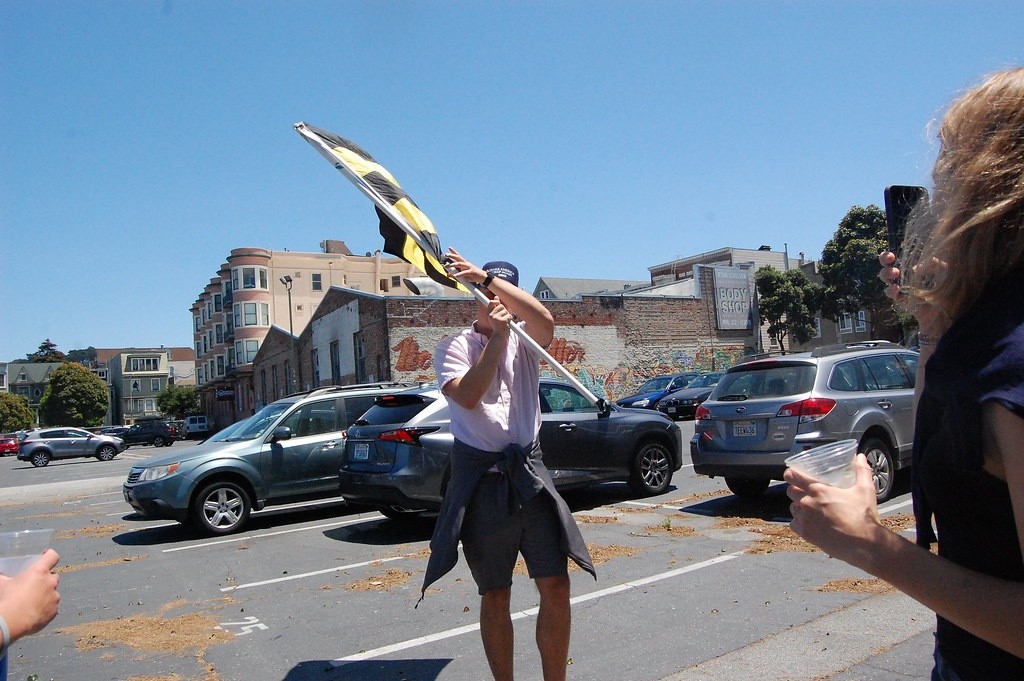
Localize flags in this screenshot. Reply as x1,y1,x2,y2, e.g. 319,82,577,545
296,121,475,293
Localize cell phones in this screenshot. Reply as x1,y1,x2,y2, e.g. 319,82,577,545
884,184,936,295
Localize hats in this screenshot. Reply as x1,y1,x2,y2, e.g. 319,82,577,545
475,261,519,287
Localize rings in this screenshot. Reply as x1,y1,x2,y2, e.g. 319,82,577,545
791,505,799,519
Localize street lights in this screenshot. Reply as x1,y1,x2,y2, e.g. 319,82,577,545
278,275,297,393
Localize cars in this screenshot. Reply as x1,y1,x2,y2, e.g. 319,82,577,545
205,407,355,443
0,427,42,458
652,372,742,421
68,418,185,438
613,370,705,410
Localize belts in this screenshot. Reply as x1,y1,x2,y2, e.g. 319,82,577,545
476,471,503,483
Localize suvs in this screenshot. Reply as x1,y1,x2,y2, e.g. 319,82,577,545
180,415,210,440
112,416,182,450
687,339,921,506
121,380,419,536
15,426,126,467
336,377,684,524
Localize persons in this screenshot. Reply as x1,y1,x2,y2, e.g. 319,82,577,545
414,248,596,681
783,68,1024,681
0,549,60,681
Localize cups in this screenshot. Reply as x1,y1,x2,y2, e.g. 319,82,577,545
0,528,54,578
784,439,858,490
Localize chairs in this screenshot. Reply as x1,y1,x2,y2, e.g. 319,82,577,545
675,380,684,388
767,378,790,394
311,418,324,434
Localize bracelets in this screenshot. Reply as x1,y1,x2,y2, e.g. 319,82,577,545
917,331,941,348
0,616,10,649
476,272,494,288
